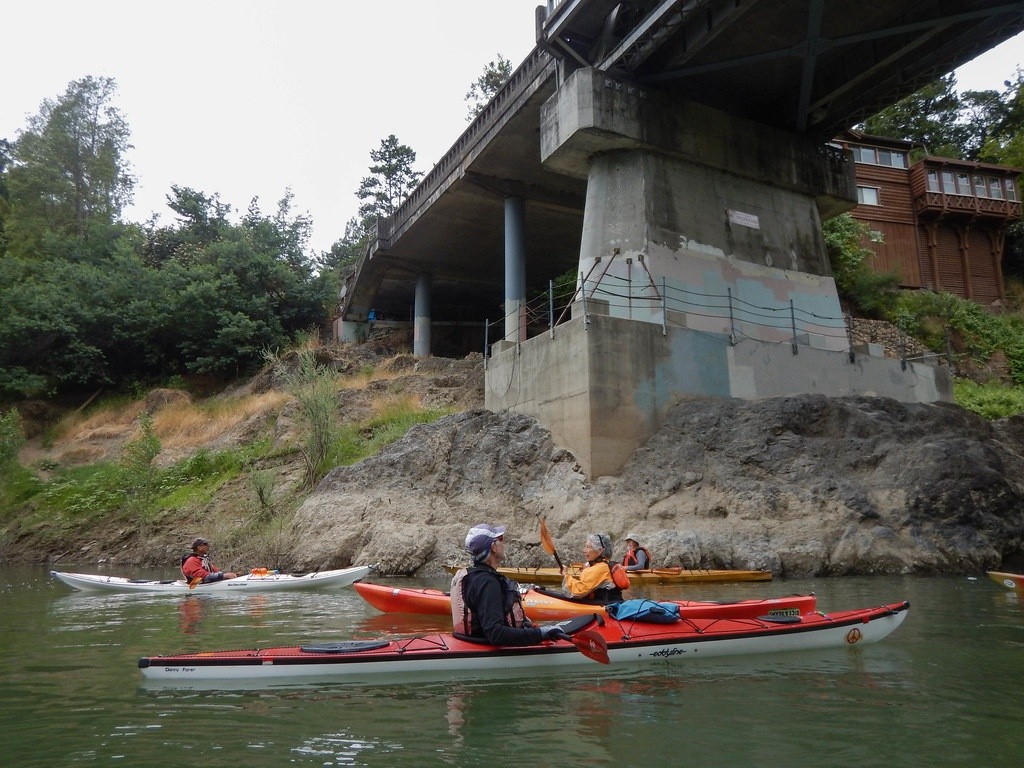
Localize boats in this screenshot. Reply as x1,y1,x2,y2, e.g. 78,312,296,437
353,578,818,623
440,561,775,586
985,567,1024,593
49,562,381,596
140,599,911,693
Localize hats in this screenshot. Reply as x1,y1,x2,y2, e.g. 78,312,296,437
465,524,506,552
192,538,209,551
624,534,640,544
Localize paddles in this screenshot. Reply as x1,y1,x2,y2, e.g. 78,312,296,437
522,620,613,667
187,577,231,590
625,566,685,577
538,511,566,573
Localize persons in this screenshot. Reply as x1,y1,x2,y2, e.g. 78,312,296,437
619,533,649,572
450,524,565,646
182,538,236,584
560,533,622,607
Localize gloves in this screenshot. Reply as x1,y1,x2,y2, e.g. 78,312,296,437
539,624,566,642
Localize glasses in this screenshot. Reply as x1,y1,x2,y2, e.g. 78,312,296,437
491,535,503,549
199,543,208,547
